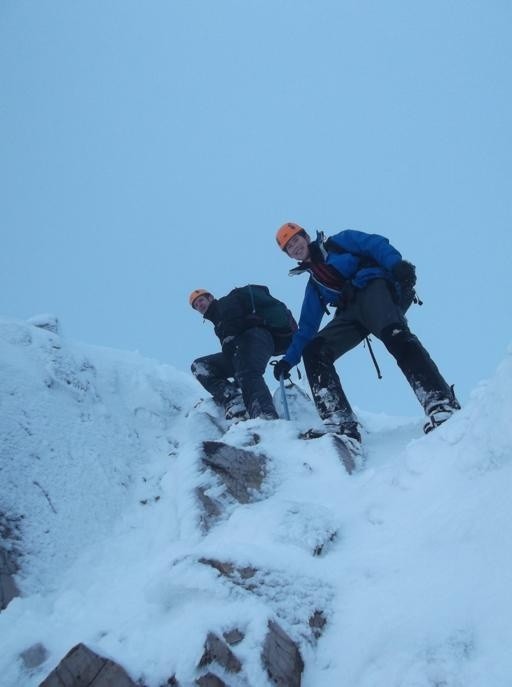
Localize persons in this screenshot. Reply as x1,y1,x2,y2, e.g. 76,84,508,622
270,223,460,440
189,289,279,419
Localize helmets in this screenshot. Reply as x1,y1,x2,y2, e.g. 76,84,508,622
189,289,211,308
276,223,304,250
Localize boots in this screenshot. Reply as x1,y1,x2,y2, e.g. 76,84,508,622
301,414,360,442
223,381,246,419
423,384,460,434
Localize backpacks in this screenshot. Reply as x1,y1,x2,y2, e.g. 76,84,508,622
226,284,298,356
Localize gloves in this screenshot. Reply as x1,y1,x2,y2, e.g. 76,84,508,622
393,261,417,284
274,360,290,380
